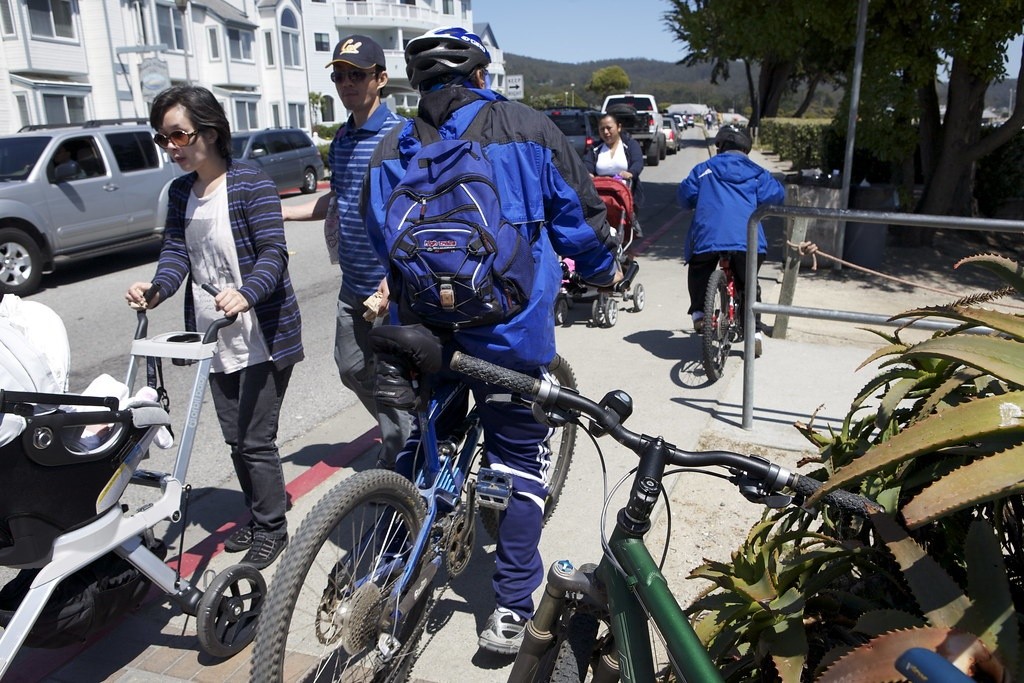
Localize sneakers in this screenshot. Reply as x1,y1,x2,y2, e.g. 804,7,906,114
478,605,528,653
223,520,254,551
238,531,289,569
369,541,412,577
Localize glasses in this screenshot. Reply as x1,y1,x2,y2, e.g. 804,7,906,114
154,129,206,150
331,71,377,82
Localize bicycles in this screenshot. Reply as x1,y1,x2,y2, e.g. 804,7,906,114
695,251,746,385
447,350,981,683
249,322,582,683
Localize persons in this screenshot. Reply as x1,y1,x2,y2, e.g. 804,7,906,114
279,36,404,504
585,114,644,238
702,110,722,129
678,123,785,355
54,145,88,179
682,111,688,128
361,27,622,653
125,87,304,567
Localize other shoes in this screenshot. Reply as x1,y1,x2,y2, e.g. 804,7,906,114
694,318,705,333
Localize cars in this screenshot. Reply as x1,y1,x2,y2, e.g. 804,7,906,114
542,93,695,166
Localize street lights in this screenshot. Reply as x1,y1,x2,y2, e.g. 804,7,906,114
565,91,569,107
571,82,576,107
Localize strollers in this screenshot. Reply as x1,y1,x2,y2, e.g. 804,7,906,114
0,281,267,681
549,171,645,328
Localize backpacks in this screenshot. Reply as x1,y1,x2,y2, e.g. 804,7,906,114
384,102,543,328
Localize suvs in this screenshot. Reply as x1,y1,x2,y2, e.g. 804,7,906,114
228,126,326,196
0,118,195,297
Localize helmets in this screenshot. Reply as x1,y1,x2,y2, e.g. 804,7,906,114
715,123,752,154
405,27,491,90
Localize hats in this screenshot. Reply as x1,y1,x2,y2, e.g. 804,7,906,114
325,35,386,69
